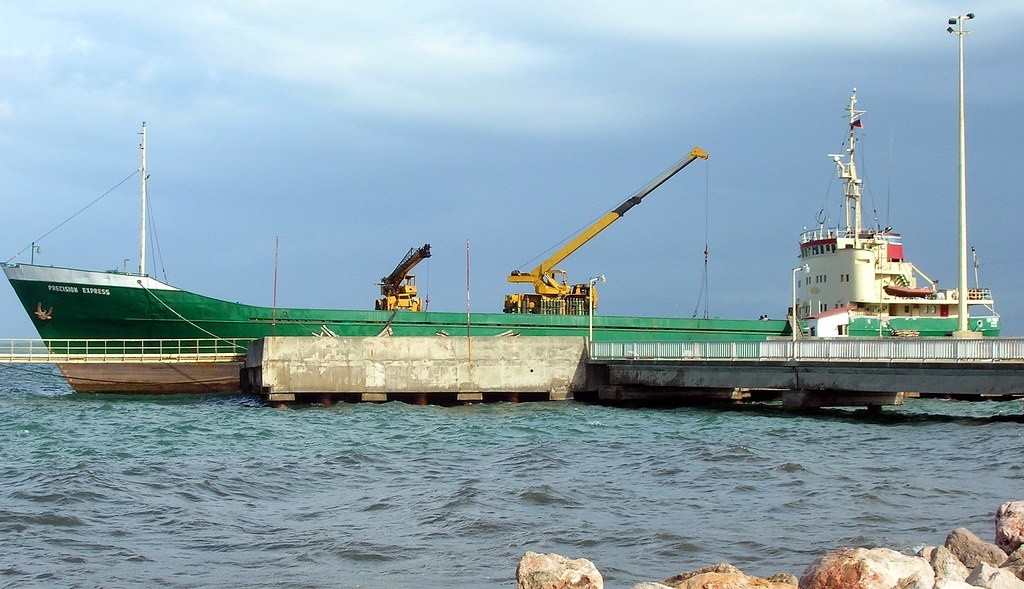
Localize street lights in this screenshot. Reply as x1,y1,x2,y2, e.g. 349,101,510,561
947,12,977,331
589,276,606,355
792,264,810,360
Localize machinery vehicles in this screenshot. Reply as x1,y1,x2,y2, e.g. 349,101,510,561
375,242,432,312
501,146,709,314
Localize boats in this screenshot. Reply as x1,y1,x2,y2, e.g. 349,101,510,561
882,282,936,298
0,114,1004,398
890,330,919,336
